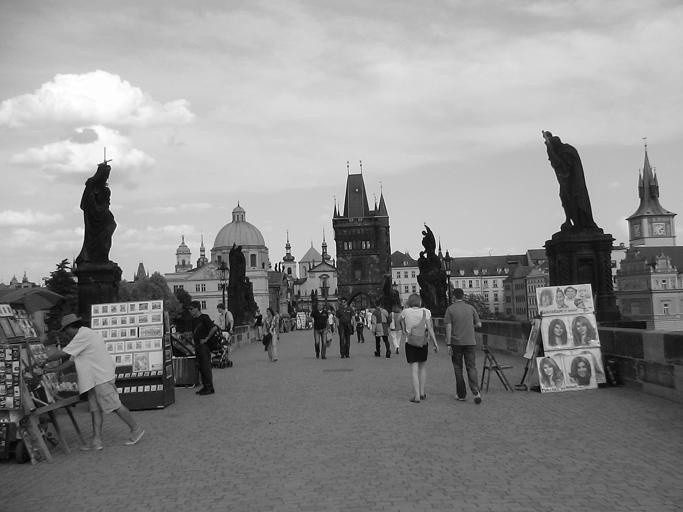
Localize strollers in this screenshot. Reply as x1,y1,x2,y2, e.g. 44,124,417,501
210,331,233,369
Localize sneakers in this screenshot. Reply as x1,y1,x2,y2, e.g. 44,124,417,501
472,387,481,404
125,423,144,445
455,394,466,401
340,353,349,358
375,347,399,357
316,353,327,359
80,441,104,451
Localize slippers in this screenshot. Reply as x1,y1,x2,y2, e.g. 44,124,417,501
410,394,427,403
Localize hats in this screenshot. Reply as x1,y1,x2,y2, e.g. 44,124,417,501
59,314,82,333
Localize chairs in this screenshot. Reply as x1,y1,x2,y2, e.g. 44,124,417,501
481,349,513,392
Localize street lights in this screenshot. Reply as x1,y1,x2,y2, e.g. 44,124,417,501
218,260,229,311
392,281,398,291
442,249,453,305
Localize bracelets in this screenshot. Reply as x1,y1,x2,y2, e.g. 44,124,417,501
446,344,452,346
230,329,232,330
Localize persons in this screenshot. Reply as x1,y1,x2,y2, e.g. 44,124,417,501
252,309,264,340
388,304,405,355
370,300,391,358
275,311,280,340
396,294,439,403
189,300,217,395
308,301,331,359
548,319,568,347
538,286,592,311
540,352,603,389
335,298,354,358
305,308,373,346
215,303,233,335
572,316,597,345
443,288,482,404
34,313,144,451
262,307,278,362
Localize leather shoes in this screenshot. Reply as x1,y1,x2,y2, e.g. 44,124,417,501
196,388,214,395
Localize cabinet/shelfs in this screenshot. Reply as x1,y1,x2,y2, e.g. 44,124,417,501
115,310,174,410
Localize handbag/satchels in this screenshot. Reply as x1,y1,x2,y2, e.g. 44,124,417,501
410,325,427,347
212,324,222,351
